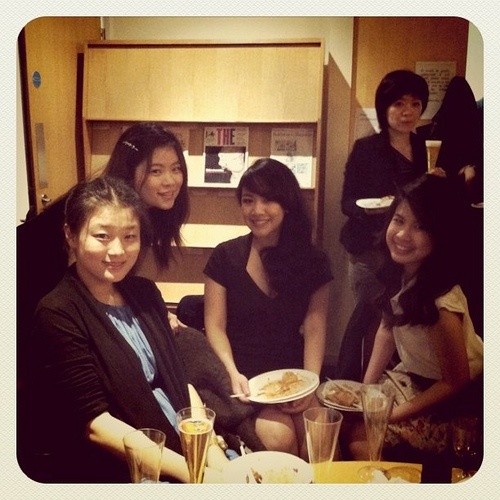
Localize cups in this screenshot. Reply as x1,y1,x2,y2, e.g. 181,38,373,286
123,427,169,484
359,384,396,462
425,138,443,172
176,407,217,484
303,405,343,463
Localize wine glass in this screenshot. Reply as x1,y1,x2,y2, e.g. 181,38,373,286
451,426,478,484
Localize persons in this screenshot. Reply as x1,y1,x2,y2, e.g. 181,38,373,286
16,122,192,354
343,172,483,463
335,69,430,384
199,157,341,463
27,176,232,482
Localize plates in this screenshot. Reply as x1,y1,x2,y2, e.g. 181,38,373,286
228,449,312,483
356,198,394,209
315,379,388,413
241,368,321,405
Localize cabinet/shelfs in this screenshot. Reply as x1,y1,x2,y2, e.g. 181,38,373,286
80,39,326,317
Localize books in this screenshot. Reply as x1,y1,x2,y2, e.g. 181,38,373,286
203,127,248,185
271,128,313,188
122,125,190,160
